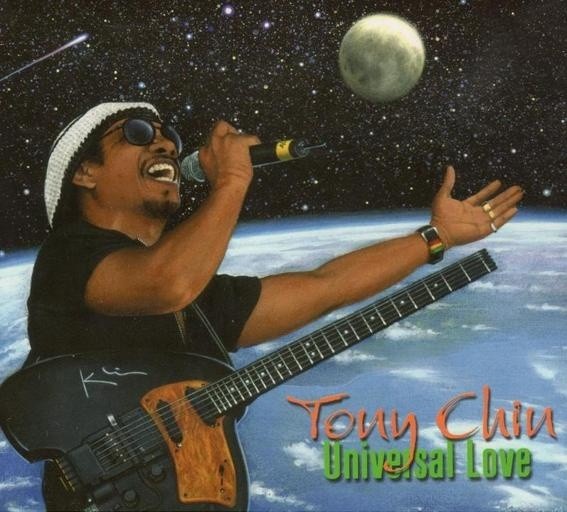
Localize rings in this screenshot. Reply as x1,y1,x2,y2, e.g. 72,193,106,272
480,202,491,212
488,211,497,220
491,224,497,233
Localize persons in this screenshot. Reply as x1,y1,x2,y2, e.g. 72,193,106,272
25,101,525,511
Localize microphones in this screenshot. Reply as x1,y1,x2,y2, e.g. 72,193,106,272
181,137,327,185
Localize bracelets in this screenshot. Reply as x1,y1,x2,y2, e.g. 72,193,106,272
416,224,444,263
